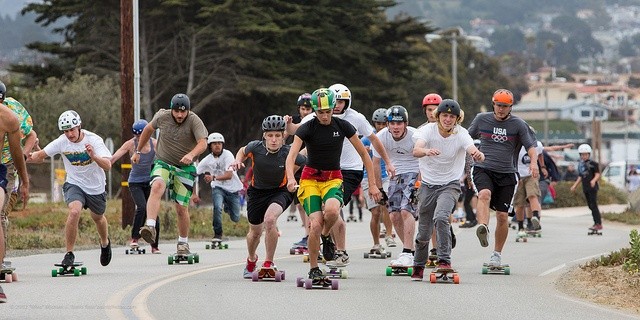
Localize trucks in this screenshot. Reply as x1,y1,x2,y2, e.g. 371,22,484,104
600,161,640,192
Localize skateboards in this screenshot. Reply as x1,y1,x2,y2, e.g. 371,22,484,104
588,227,602,235
168,253,199,265
482,263,510,275
509,223,543,242
252,266,285,282
290,242,308,254
206,241,228,249
430,269,459,284
386,265,413,276
326,260,346,279
304,251,326,263
0,266,17,283
51,262,87,277
126,247,145,254
296,276,338,290
364,251,391,259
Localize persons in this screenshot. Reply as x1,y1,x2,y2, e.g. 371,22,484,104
467,87,539,268
459,147,480,229
417,93,458,262
281,82,397,269
283,86,381,280
282,92,315,249
369,105,422,268
627,165,637,184
129,93,208,258
31,109,112,265
0,81,39,270
113,118,160,255
228,115,307,280
515,126,549,238
569,144,603,231
410,98,485,283
349,182,364,223
473,138,481,150
526,149,561,231
359,108,398,254
193,132,242,243
0,101,31,304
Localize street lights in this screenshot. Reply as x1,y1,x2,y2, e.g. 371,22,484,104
425,26,482,103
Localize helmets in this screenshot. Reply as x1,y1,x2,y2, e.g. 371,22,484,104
328,84,352,119
384,105,409,125
310,88,336,111
578,144,592,153
492,89,513,106
58,110,81,130
422,93,442,108
437,99,460,115
262,115,285,132
207,132,225,145
132,119,148,135
297,93,311,106
372,108,387,122
170,94,190,110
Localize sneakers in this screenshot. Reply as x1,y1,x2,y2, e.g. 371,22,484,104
326,250,350,266
531,217,539,230
130,239,138,246
430,248,437,255
390,253,414,267
385,237,397,247
518,229,526,237
262,261,274,268
294,235,309,246
308,267,322,279
489,253,502,266
320,234,335,261
177,242,191,255
212,235,222,242
139,225,156,244
62,252,74,265
411,265,424,281
100,238,111,266
243,255,258,278
435,258,451,270
476,224,488,247
152,248,161,254
589,224,603,230
